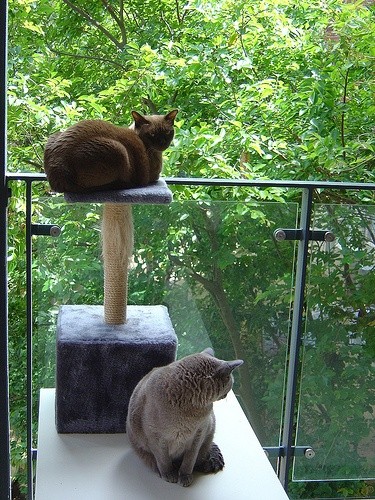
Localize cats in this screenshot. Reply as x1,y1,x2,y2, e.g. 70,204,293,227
125,348,244,487
44,109,179,195
